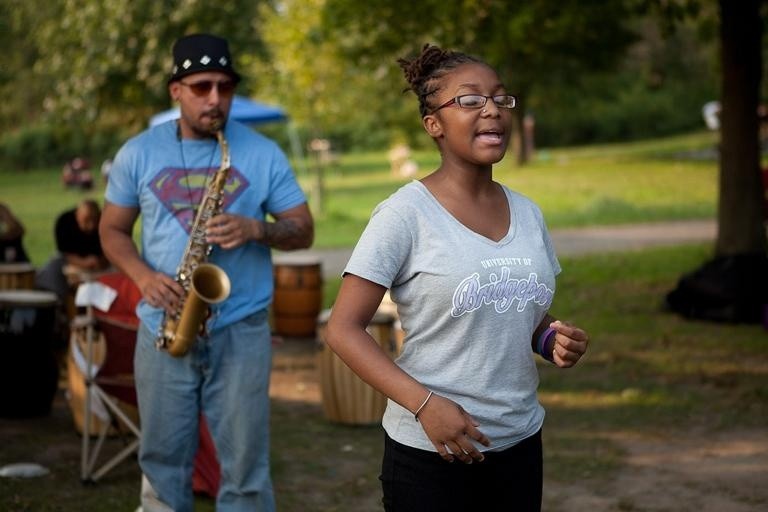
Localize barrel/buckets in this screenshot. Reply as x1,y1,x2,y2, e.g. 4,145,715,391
0,262,34,291
273,264,322,336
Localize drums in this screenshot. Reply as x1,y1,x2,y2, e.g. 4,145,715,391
65,315,136,435
272,254,323,341
1,265,37,290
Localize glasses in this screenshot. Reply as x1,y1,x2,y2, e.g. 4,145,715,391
431,95,517,114
182,80,238,97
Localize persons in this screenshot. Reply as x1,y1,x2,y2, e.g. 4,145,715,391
322,41,592,512
98,30,316,512
32,198,119,297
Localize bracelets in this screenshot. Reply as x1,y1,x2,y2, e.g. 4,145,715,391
536,323,556,358
414,390,434,424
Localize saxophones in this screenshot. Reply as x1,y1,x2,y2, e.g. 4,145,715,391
154,117,233,358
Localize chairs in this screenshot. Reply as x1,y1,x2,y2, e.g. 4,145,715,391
81,274,141,486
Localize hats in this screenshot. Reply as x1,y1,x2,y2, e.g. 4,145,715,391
168,34,240,85
